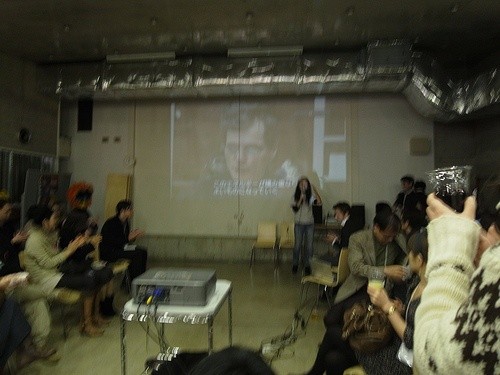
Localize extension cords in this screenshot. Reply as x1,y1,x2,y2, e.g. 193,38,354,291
262,343,276,357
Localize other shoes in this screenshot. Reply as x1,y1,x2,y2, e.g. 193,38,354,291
53,288,81,302
293,264,298,272
305,267,310,276
34,347,62,365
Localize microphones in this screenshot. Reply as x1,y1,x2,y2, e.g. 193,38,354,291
300,190,305,206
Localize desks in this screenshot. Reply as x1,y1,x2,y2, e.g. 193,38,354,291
120,279,233,375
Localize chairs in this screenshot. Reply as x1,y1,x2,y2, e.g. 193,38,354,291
19,220,349,341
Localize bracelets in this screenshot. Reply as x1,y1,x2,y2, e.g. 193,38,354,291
385,303,397,315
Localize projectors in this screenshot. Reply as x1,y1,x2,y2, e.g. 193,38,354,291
132,268,217,306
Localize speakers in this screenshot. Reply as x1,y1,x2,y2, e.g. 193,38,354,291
77,98,93,132
352,205,365,227
313,206,322,224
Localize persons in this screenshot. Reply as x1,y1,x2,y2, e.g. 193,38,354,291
300,174,500,375
194,108,308,221
0,184,149,375
291,174,323,277
188,340,277,375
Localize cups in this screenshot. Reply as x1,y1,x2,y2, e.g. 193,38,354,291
367,266,386,291
424,165,470,214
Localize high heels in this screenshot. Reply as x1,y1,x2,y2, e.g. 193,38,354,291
80,320,104,337
94,315,111,327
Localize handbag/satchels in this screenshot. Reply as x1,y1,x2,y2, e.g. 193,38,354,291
344,303,388,353
